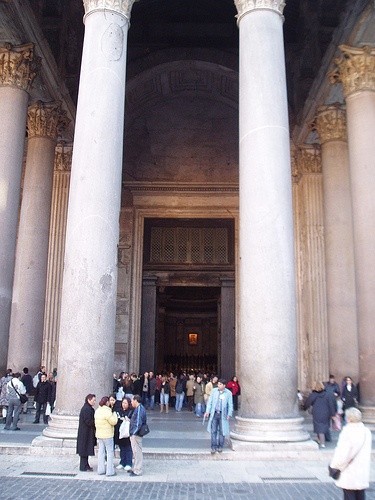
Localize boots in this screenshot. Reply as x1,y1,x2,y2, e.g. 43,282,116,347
166,404,169,414
160,405,163,414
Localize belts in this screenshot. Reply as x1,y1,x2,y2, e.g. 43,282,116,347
215,410,222,414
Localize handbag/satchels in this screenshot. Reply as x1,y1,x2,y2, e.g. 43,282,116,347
45,403,51,415
332,414,342,432
2,408,7,418
329,466,340,479
33,401,36,409
119,418,131,439
137,425,150,436
19,394,28,404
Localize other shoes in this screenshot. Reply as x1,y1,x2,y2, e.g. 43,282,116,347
218,447,222,452
14,427,20,430
86,468,93,472
128,470,142,476
211,448,216,454
124,465,131,470
116,464,124,469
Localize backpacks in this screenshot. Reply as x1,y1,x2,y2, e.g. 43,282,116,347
33,371,43,388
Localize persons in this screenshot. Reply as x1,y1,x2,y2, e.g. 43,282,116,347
33,374,51,424
113,367,241,418
297,381,335,448
33,366,57,420
329,407,372,500
325,375,341,429
76,394,147,477
204,378,233,453
0,367,33,424
4,373,26,430
340,377,359,411
